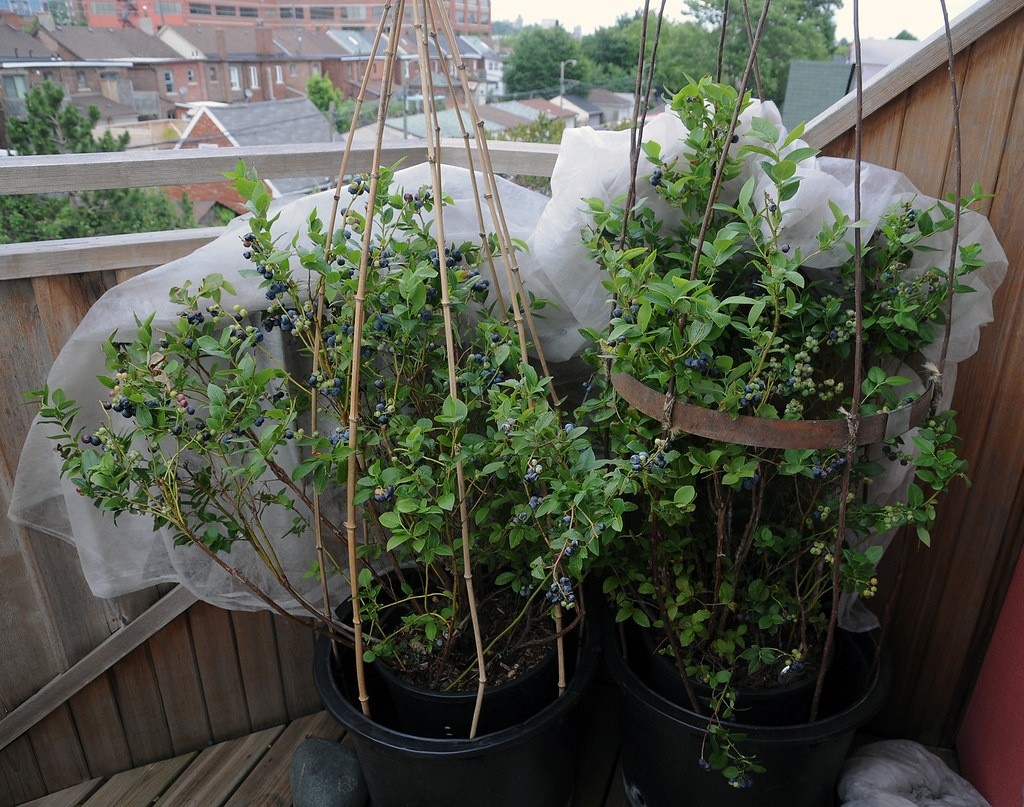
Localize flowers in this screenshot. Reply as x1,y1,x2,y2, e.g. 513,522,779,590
20,73,1003,787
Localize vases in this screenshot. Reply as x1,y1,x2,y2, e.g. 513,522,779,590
317,571,888,807
